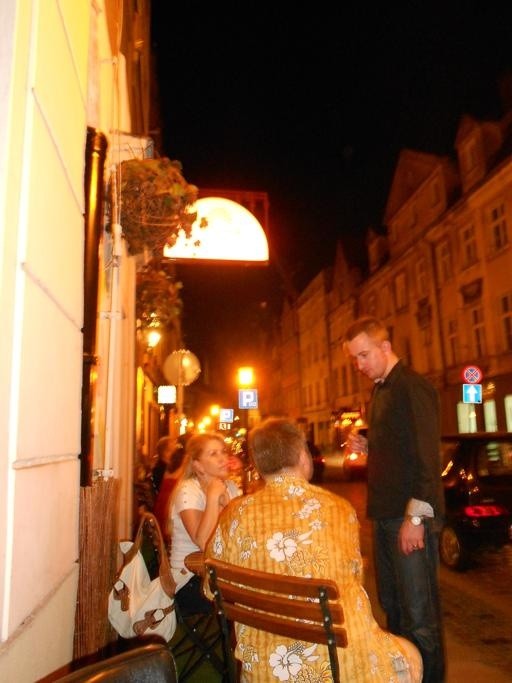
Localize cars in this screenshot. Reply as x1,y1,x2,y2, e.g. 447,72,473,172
438,430,511,573
306,440,326,483
341,426,371,483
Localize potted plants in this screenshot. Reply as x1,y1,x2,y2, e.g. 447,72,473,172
105,154,209,328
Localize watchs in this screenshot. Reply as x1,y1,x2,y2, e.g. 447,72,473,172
405,515,431,527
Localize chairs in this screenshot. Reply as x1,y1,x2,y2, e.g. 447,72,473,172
133,481,349,683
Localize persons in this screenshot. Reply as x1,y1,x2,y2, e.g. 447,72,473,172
147,430,265,559
203,416,425,681
343,317,446,681
162,432,243,682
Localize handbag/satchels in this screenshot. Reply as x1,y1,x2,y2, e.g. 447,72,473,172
106,512,177,644
